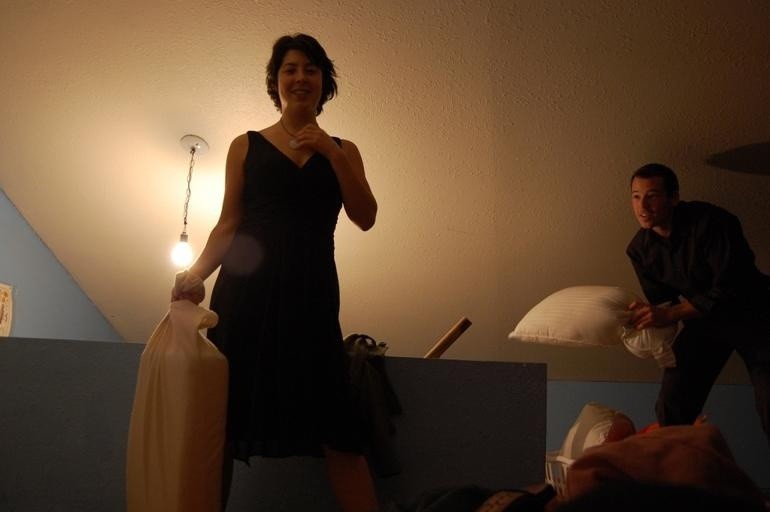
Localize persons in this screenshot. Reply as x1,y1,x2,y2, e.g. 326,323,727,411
171,29,382,512
623,161,770,451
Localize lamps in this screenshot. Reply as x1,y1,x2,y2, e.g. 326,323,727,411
170,134,210,268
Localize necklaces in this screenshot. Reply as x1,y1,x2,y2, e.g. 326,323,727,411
276,117,301,150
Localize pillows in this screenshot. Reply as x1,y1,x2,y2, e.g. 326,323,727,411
507,284,685,369
